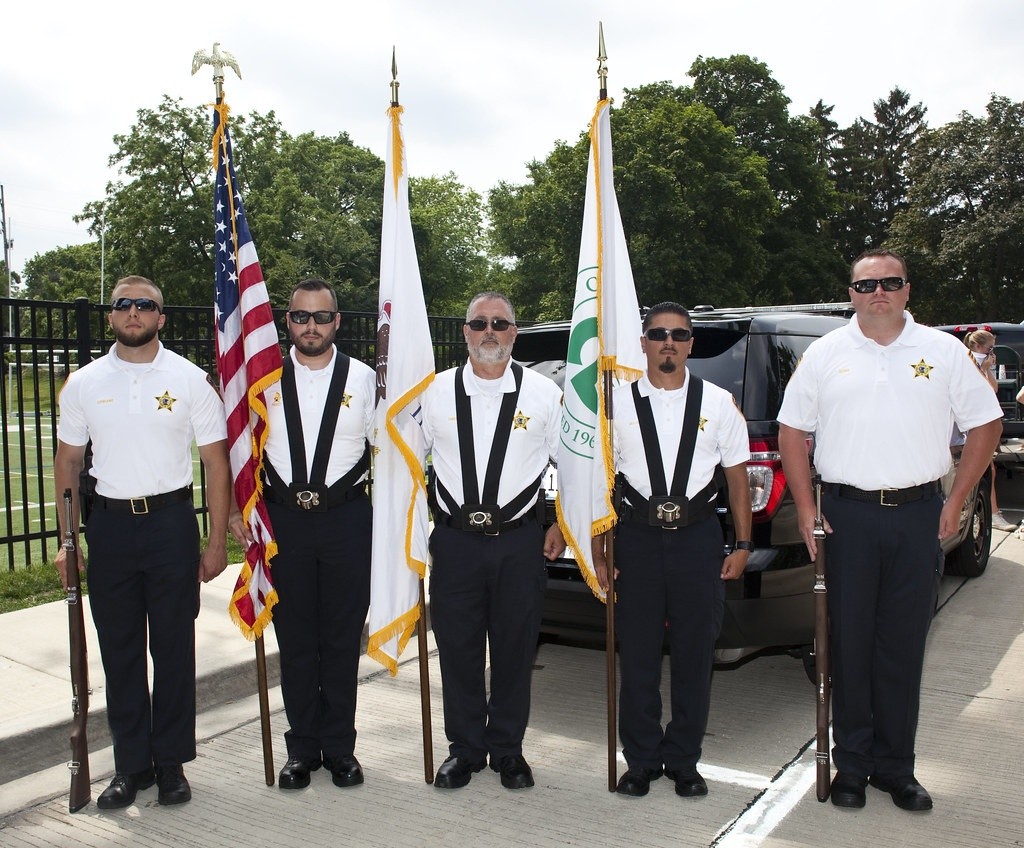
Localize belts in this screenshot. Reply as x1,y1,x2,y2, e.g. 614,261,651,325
822,479,944,507
261,481,366,513
437,505,538,536
620,500,716,525
91,483,192,514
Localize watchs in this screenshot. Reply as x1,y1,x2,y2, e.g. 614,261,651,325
734,540,754,552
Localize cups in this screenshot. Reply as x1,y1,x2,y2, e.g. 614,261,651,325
999,365,1006,379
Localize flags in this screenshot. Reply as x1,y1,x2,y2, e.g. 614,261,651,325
211,92,283,641
367,106,435,677
555,99,647,605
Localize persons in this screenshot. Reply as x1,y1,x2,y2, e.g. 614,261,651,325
778,251,1003,812
53,276,232,810
421,293,564,790
228,276,377,788
591,302,752,797
949,329,1017,531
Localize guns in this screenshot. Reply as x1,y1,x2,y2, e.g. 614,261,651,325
812,472,831,803
62,486,92,813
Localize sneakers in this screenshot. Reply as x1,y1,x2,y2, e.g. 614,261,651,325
991,511,1017,530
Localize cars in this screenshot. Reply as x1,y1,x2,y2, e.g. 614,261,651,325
924,320,1024,465
510,306,997,693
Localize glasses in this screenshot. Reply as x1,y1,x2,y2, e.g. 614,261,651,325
111,298,163,312
286,310,341,324
981,345,993,354
850,276,906,293
641,328,691,341
465,318,517,331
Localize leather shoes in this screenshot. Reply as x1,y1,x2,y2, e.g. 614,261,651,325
278,753,321,788
96,770,154,809
489,752,534,788
153,765,191,805
322,752,364,785
434,752,487,788
664,760,708,795
830,771,867,807
868,770,932,809
615,764,663,795
